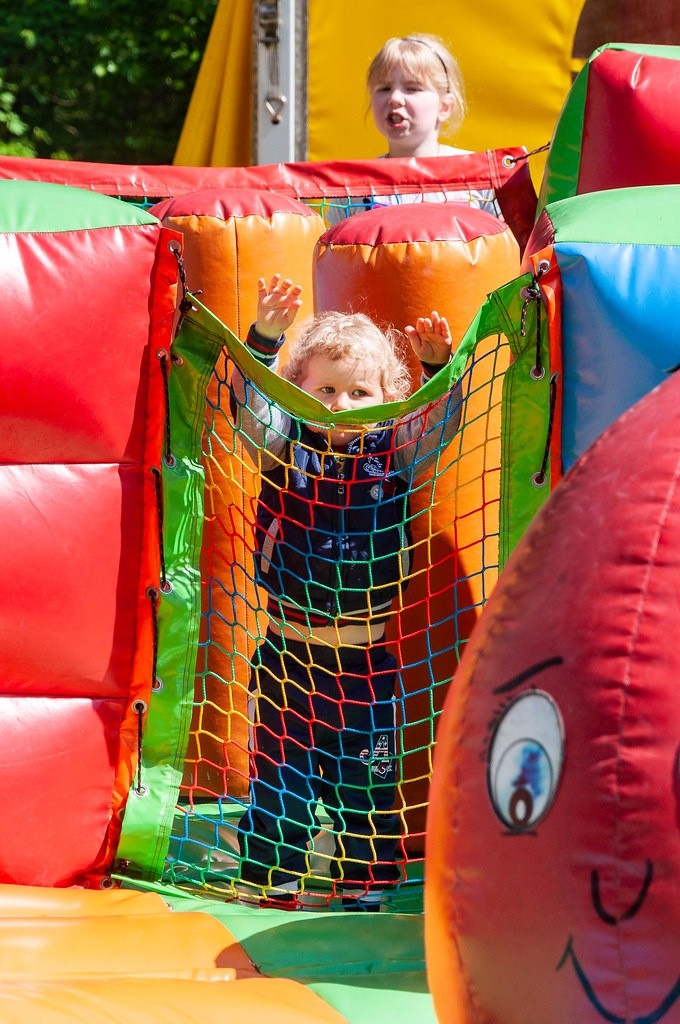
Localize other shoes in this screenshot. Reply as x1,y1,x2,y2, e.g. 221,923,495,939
261,895,300,912
341,899,382,913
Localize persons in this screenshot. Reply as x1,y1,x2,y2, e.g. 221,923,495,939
230,273,462,912
326,35,504,223
